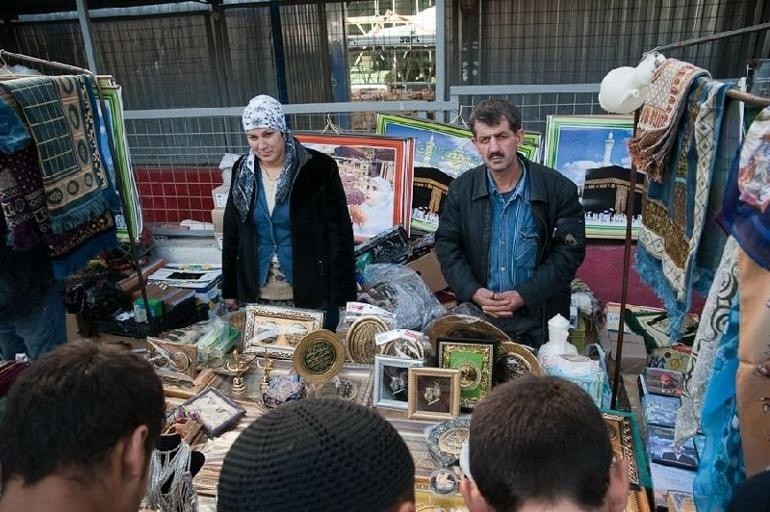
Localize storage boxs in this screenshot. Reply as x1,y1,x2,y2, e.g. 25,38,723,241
605,301,699,335
147,262,223,320
211,168,232,252
65,309,82,344
567,317,586,356
406,248,449,294
96,331,155,352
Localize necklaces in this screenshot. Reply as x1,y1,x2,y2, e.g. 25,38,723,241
145,438,198,512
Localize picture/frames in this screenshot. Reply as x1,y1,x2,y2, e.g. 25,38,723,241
602,413,639,491
244,305,323,360
180,386,246,436
372,336,499,422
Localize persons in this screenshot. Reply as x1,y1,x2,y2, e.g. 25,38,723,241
433,98,586,350
460,374,628,512
222,93,358,334
0,339,166,511
1,101,67,360
342,174,393,237
